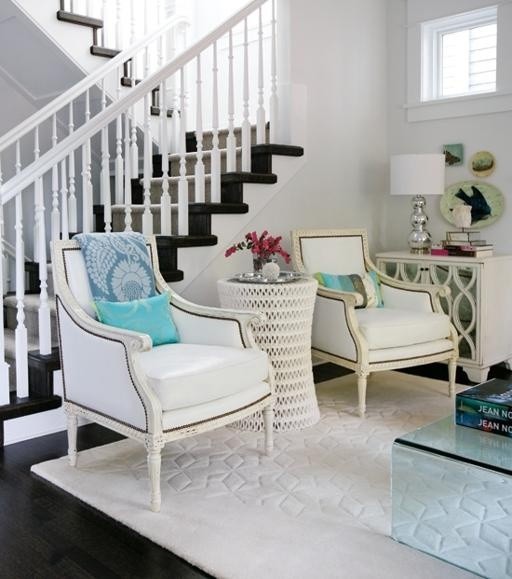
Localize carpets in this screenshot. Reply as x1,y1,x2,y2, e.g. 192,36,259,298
29,371,490,578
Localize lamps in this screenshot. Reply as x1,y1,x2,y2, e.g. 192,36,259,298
390,155,445,255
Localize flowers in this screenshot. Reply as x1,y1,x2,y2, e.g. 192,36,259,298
220,230,293,260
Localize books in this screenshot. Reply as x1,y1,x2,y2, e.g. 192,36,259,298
429,230,494,258
455,410,512,437
456,376,512,422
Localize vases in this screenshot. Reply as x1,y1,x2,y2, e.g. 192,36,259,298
253,254,270,276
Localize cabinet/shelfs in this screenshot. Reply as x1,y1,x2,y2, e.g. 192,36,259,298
375,249,512,383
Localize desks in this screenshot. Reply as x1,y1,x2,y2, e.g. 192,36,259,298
215,272,326,430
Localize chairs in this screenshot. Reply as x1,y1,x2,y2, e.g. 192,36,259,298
289,229,454,417
52,233,276,514
